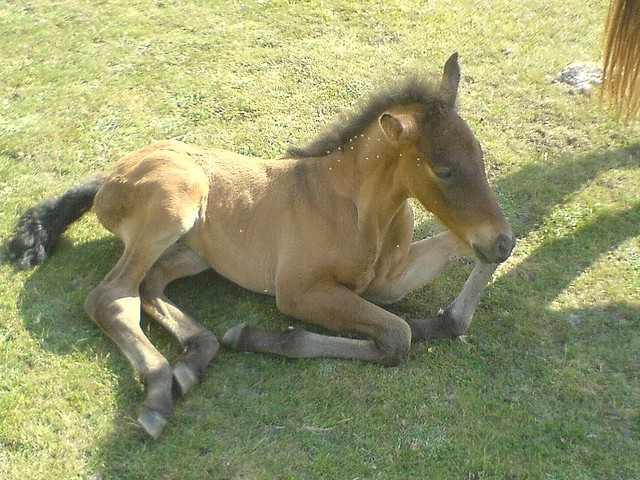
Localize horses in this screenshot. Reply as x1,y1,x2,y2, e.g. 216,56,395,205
7,50,514,440
